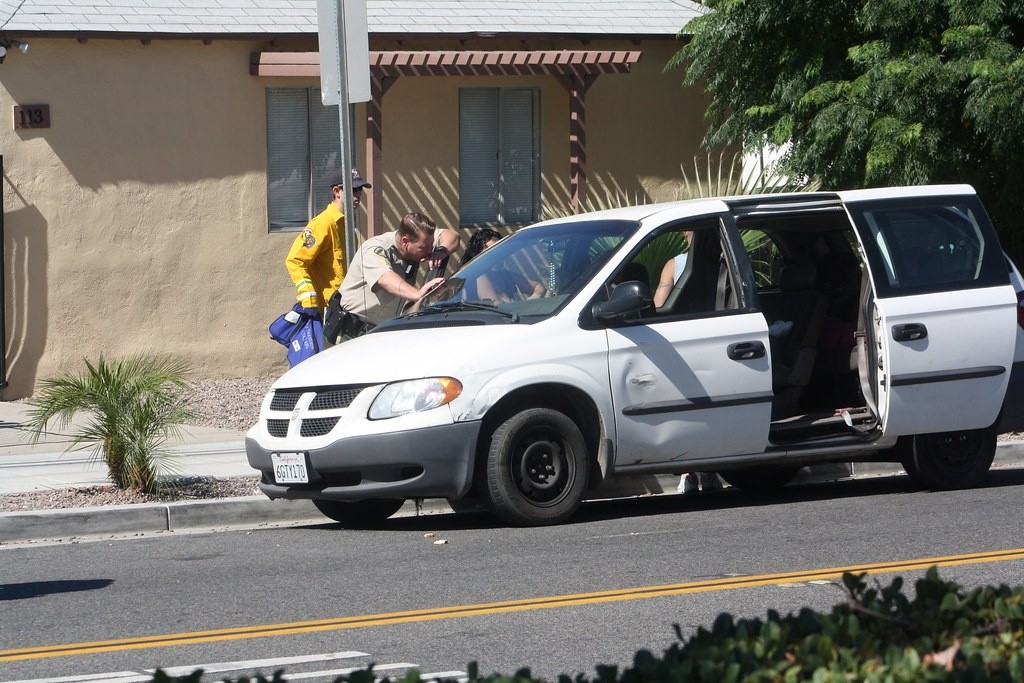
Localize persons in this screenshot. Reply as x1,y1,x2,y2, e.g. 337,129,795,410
652,226,731,312
325,210,462,346
286,165,373,350
457,228,546,302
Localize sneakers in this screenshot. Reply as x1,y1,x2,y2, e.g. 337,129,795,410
676,471,723,492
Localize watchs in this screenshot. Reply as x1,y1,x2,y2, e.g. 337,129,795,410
434,245,449,254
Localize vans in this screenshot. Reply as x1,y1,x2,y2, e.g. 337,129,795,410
243,180,1024,524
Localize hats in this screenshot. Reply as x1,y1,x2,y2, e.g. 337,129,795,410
330,166,372,188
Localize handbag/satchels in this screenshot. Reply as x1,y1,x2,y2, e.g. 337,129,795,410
324,289,350,346
268,299,324,371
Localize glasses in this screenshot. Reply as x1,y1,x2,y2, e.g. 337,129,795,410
338,184,364,192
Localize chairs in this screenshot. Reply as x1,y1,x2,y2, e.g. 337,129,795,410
581,251,657,319
772,257,827,417
